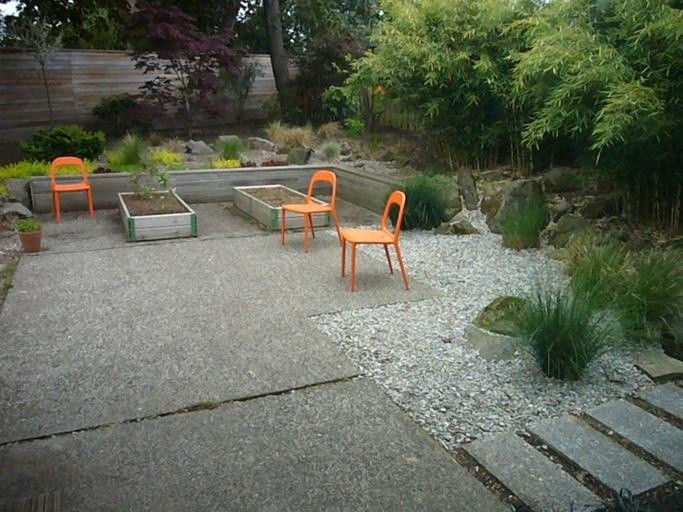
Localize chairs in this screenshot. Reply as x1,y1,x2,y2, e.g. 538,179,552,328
281,170,343,253
50,156,93,221
340,189,410,293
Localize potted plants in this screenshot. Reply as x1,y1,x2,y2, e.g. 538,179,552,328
508,275,623,383
118,159,197,242
15,216,44,253
